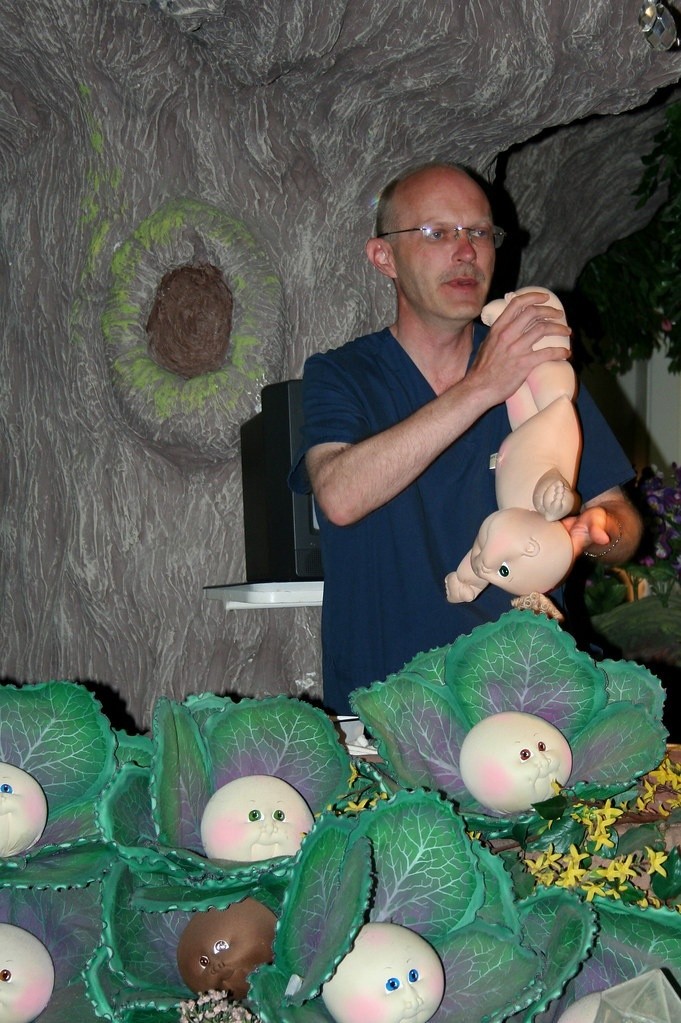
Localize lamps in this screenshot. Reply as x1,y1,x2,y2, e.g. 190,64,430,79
637,0,681,53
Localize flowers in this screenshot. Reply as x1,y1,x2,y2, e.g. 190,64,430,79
326,752,681,913
567,462,680,615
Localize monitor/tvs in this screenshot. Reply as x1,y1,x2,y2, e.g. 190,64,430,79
241,379,325,582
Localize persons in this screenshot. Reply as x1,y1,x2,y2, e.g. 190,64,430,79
298,165,643,744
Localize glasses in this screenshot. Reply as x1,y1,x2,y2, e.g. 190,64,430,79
377,223,507,249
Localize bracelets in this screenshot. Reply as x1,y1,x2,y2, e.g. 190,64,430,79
583,511,623,561
